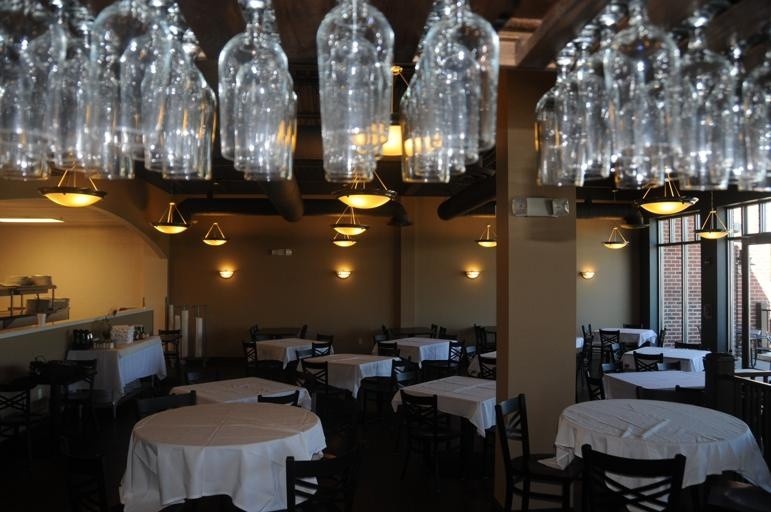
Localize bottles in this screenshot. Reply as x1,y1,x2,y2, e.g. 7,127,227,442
93,338,115,350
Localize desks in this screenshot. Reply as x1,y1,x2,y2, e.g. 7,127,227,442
559,400,749,510
132,402,321,511
400,375,496,469
170,376,308,403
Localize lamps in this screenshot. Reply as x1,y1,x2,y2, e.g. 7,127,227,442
475,217,497,248
150,182,193,234
381,63,409,157
38,170,106,208
331,206,369,235
218,270,235,279
694,190,731,239
335,270,352,278
601,188,629,250
465,271,480,279
332,204,357,247
201,214,229,246
634,172,699,215
581,272,595,278
333,170,396,209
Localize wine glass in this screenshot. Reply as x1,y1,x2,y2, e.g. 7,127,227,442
315,1,396,184
218,0,298,182
1,1,217,180
530,0,770,190
400,1,501,183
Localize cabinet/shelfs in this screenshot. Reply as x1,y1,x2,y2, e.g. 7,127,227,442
0,282,70,324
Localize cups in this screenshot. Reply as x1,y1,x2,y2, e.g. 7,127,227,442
37,312,46,327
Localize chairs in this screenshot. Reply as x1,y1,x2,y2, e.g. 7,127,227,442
286,450,354,512
242,321,497,429
495,393,583,510
580,444,687,511
399,389,467,474
576,322,712,399
3,310,181,511
635,384,709,409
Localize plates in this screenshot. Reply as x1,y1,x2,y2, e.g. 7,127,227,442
9,274,52,287
